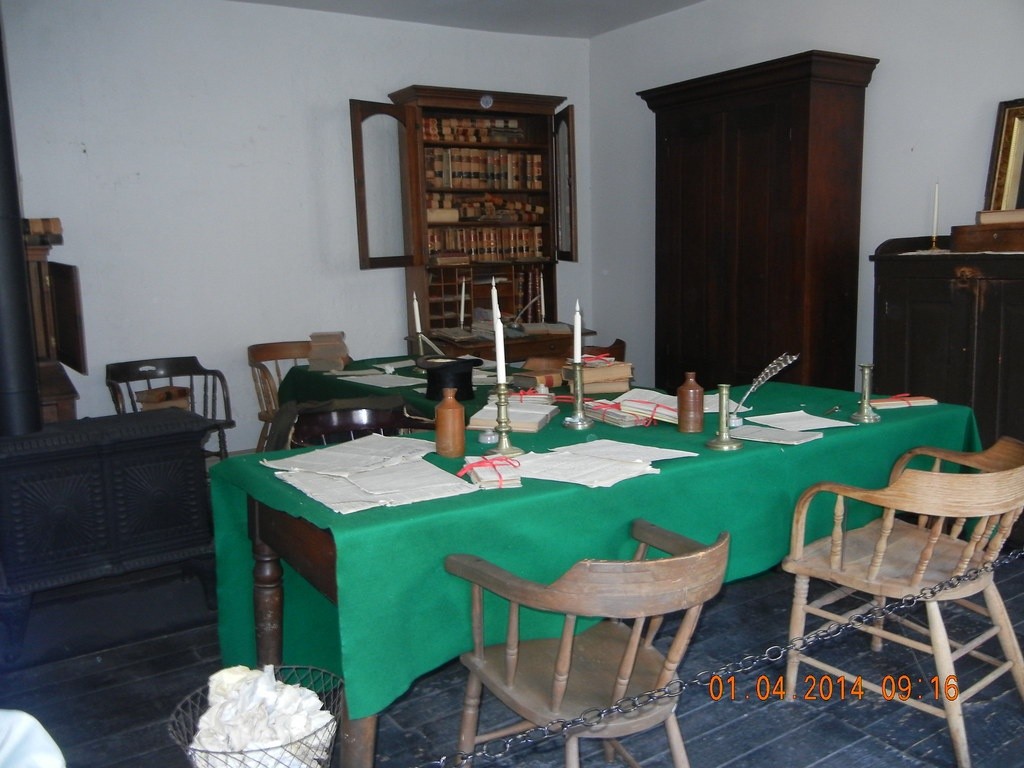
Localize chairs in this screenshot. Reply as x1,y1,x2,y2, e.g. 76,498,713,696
248,340,355,452
106,355,237,488
444,516,729,768
780,435,1024,768
564,337,626,363
263,396,437,459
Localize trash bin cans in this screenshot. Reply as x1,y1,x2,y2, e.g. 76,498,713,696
166,666,345,768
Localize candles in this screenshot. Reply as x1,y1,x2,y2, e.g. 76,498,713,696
413,289,421,333
573,298,582,363
494,304,507,383
460,276,466,321
932,183,939,236
491,276,499,332
540,272,545,316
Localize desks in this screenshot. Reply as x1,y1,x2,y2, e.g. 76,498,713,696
275,355,568,429
238,380,973,768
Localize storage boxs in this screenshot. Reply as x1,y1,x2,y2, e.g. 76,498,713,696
951,223,1024,252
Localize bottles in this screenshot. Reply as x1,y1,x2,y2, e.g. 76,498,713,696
677,371,704,434
435,388,464,458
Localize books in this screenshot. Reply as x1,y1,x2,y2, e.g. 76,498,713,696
975,209,1024,225
422,118,548,265
562,354,634,392
717,425,823,445
512,370,562,388
462,452,523,490
583,387,678,428
467,400,560,433
488,393,555,405
136,386,190,411
22,218,63,245
307,331,348,372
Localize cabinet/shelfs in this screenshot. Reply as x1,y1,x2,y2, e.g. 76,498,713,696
349,84,599,364
636,50,880,398
22,218,89,427
866,234,1024,561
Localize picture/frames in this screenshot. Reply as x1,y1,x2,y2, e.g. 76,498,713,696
984,97,1024,208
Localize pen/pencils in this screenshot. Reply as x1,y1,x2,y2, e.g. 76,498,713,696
824,404,841,415
